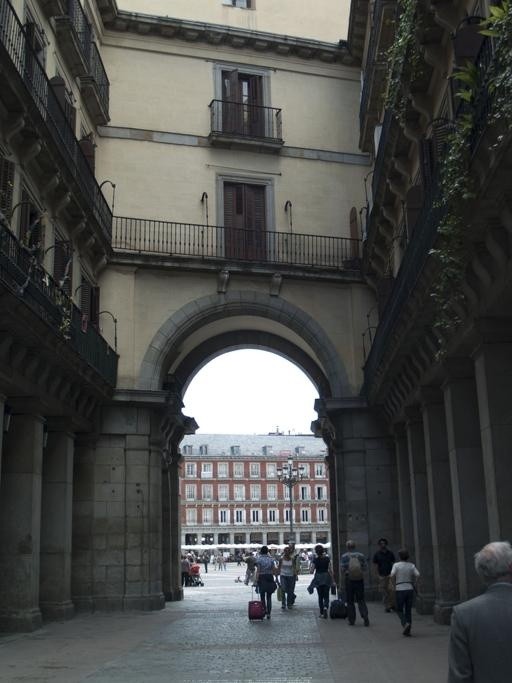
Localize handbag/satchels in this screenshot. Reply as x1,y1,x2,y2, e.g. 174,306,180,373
330,595,347,619
278,587,284,601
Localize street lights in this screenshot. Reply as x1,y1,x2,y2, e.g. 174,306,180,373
277,456,304,542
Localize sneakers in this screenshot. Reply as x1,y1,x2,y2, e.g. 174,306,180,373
402,623,411,634
281,605,293,609
348,617,370,626
319,607,328,618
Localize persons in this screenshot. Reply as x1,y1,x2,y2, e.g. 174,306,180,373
445,540,511,682
182,547,227,587
339,540,370,626
372,538,398,613
252,546,280,619
386,549,419,637
275,547,297,609
233,543,333,586
307,545,336,618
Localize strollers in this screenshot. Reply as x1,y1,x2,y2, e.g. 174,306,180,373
188,564,203,586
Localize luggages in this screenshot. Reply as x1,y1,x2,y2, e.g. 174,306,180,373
248,584,266,622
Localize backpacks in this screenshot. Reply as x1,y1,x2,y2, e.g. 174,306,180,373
348,552,363,582
